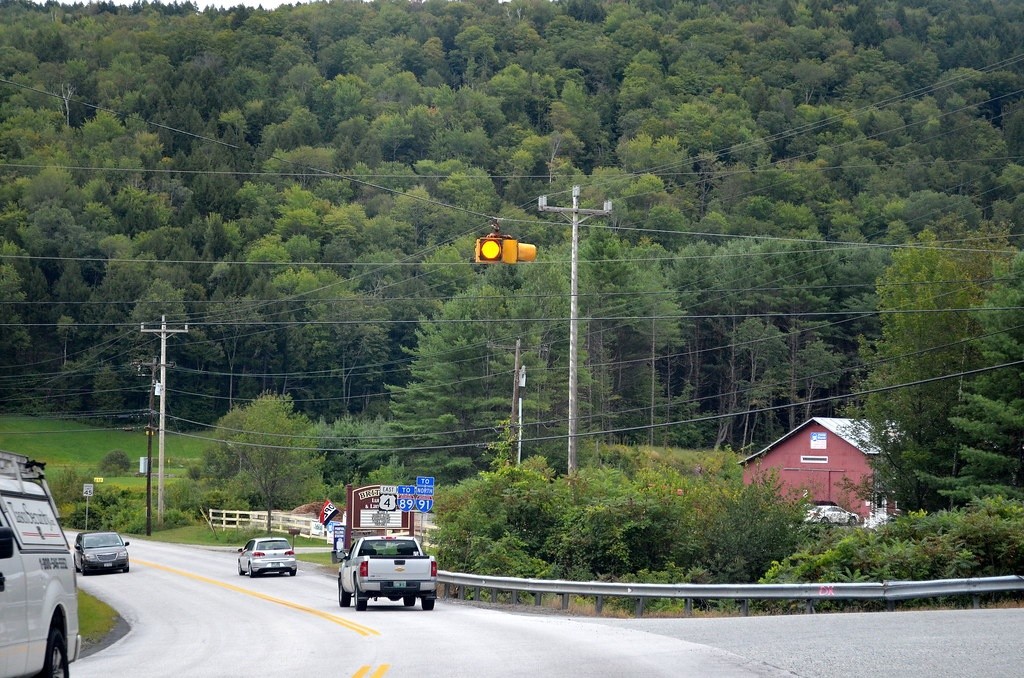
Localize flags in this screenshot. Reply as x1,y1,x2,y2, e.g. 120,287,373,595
319,500,340,526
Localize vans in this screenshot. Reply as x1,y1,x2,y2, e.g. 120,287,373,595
0,450,84,678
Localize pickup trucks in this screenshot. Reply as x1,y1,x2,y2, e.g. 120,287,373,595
339,535,438,611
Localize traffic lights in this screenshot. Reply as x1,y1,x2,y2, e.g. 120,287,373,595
475,236,537,264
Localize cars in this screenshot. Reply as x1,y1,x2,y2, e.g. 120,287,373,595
74,531,130,575
805,506,860,527
238,537,298,578
